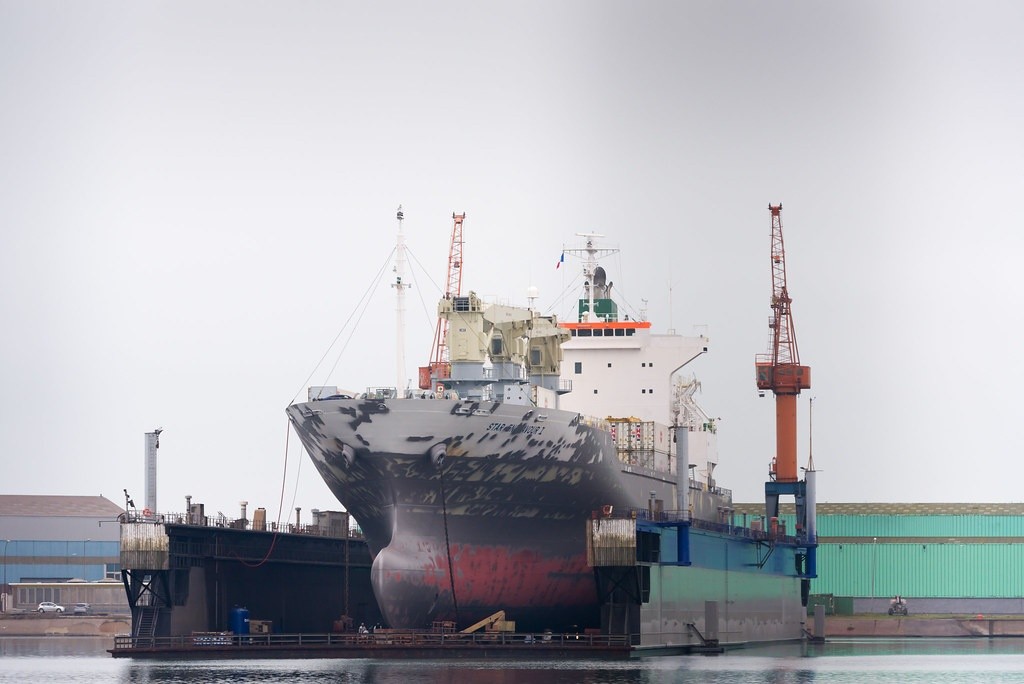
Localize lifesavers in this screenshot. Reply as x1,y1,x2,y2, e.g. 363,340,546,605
142,508,152,517
603,504,613,515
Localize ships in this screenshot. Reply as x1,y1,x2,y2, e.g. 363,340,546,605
284,202,735,631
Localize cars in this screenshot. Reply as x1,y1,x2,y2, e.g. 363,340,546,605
74,602,93,616
37,601,66,613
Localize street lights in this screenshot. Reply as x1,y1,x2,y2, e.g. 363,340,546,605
4,539,11,615
83,538,91,604
